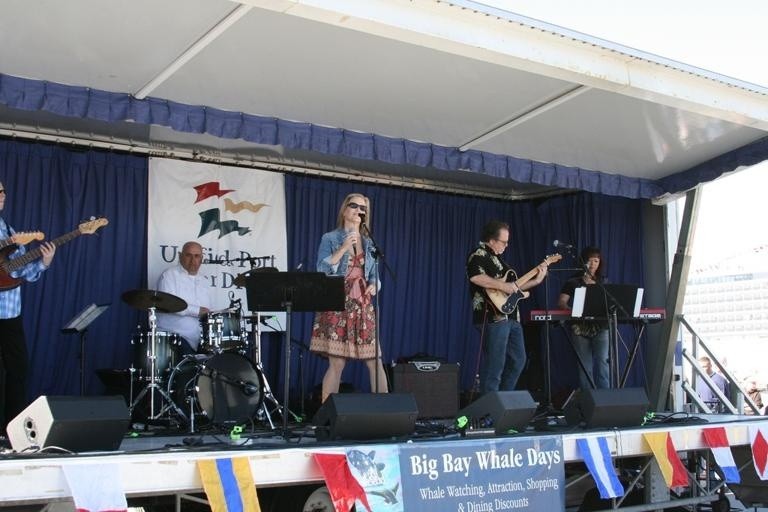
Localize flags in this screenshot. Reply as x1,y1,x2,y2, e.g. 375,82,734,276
642,431,689,488
576,437,624,500
748,422,768,481
62,464,127,512
703,427,740,484
197,456,261,512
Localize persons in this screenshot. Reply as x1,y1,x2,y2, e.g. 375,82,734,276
155,242,211,356
557,248,610,390
742,377,763,416
464,220,548,397
0,182,57,450
309,193,388,404
697,356,730,414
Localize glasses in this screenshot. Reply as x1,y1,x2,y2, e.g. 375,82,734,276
348,203,367,211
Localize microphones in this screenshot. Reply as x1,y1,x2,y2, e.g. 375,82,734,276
203,258,224,264
358,212,367,228
552,240,573,249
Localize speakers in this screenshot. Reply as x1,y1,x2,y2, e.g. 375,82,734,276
311,392,419,441
455,390,537,434
392,362,462,420
564,387,651,428
7,394,133,456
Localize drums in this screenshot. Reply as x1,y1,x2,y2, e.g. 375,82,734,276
168,354,263,429
200,308,244,352
135,330,179,383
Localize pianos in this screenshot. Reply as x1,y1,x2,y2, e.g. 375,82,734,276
523,307,667,323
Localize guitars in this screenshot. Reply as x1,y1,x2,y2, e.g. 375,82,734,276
484,253,561,318
1,215,110,291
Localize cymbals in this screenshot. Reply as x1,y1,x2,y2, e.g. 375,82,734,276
233,268,279,287
120,289,188,313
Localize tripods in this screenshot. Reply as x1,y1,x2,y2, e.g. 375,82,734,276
252,338,285,430
130,329,188,424
